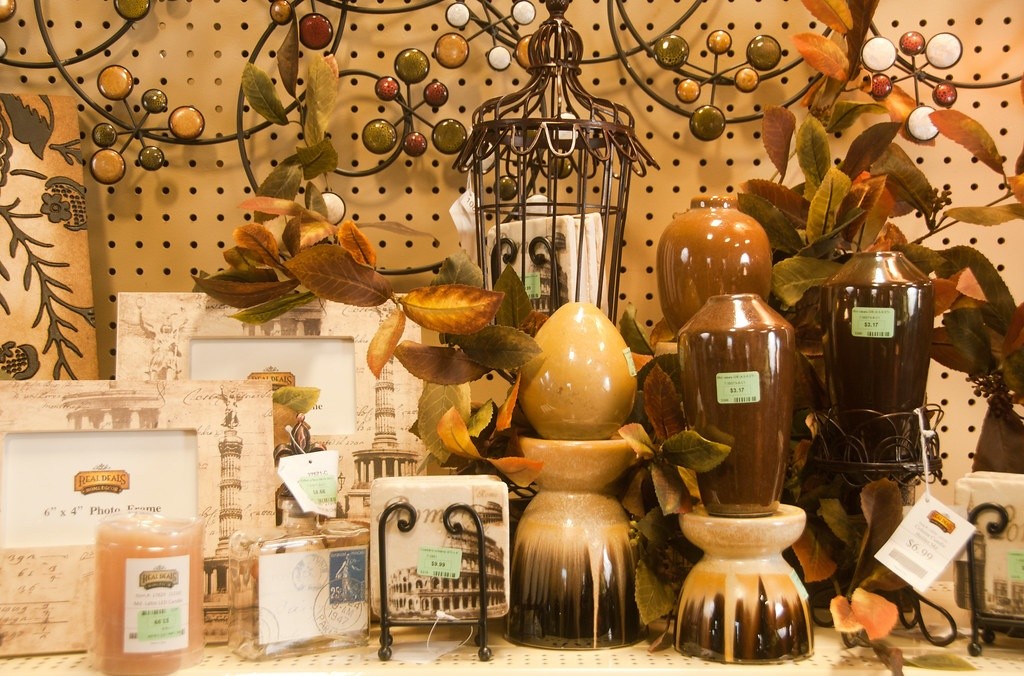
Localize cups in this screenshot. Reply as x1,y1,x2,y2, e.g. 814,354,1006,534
94,509,204,676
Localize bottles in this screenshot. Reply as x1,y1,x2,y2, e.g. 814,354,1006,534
226,495,372,663
